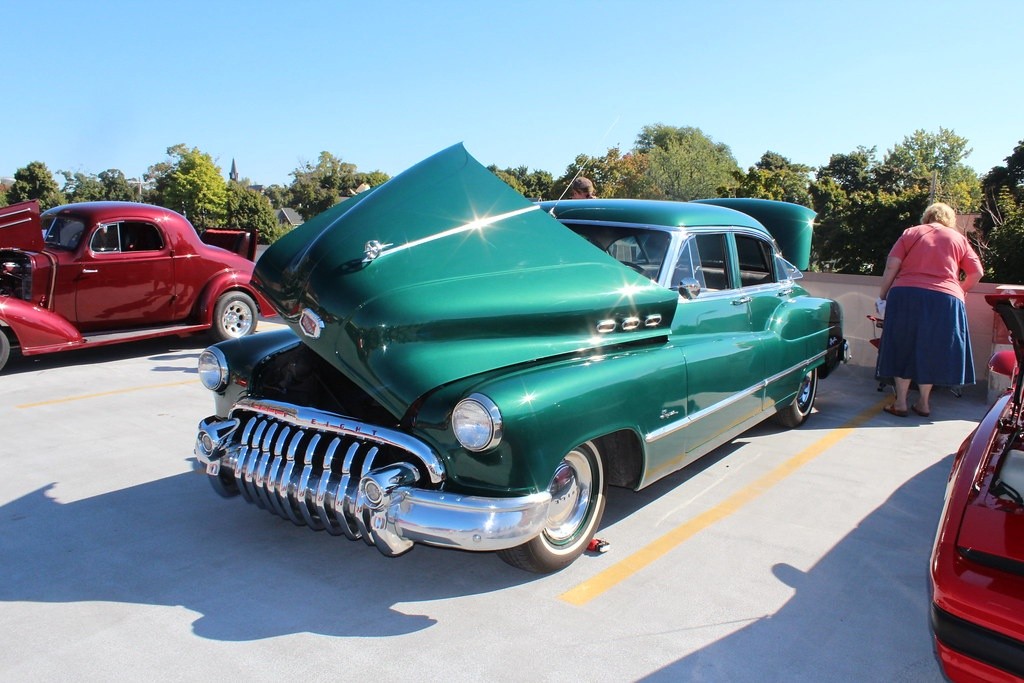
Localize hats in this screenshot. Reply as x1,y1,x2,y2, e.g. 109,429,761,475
573,177,594,192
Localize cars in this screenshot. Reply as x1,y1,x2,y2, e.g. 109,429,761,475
0,198,279,375
929,285,1024,683
195,141,853,575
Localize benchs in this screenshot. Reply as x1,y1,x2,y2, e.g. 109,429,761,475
647,268,770,290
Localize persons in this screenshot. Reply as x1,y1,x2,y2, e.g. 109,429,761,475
876,201,985,417
572,177,598,199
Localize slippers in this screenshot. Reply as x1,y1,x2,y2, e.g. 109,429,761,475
883,404,908,417
911,403,929,417
